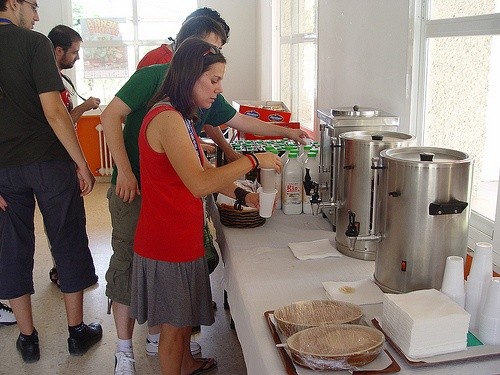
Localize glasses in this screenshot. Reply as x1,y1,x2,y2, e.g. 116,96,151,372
203,46,221,56
27,1,40,12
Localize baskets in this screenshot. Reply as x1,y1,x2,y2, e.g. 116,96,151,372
215,201,267,229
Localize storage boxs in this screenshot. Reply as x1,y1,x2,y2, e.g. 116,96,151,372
228,100,300,140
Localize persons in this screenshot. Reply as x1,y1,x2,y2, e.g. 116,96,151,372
137,6,231,311
0,0,103,363
43,25,101,285
130,37,283,375
101,17,310,374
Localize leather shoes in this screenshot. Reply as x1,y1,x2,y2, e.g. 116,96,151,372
68,322,103,355
16,332,40,362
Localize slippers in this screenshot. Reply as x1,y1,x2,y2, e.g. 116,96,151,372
190,357,219,373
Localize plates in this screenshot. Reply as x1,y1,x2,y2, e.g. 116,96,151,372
218,204,266,228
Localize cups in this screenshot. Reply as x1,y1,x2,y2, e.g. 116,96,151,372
256,169,279,218
440,242,500,346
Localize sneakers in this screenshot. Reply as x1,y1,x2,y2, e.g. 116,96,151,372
145,338,202,355
114,348,135,374
1,302,17,326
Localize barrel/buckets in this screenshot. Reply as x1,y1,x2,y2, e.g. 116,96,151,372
311,130,416,260
355,147,475,294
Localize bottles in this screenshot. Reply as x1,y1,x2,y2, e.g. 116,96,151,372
229,138,320,215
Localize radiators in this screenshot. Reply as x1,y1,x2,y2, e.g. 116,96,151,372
96,123,124,177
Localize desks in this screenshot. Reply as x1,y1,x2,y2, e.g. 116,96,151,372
207,194,500,375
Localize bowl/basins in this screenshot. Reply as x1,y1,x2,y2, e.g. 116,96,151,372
275,299,384,369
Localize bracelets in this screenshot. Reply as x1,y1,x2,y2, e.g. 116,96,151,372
244,151,259,170
234,186,251,211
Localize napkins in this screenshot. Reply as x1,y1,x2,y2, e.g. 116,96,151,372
289,239,342,260
383,289,471,358
322,278,383,304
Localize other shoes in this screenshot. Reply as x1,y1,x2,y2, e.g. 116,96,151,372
49,267,61,288
